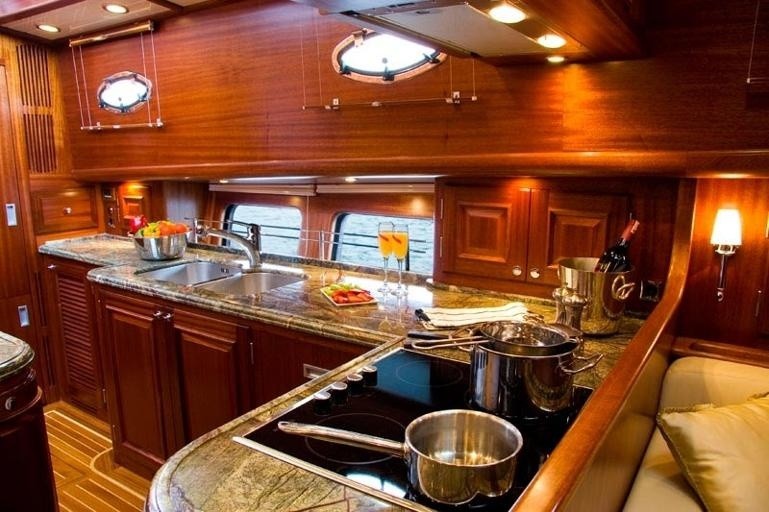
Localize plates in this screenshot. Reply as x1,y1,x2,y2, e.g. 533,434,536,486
319,284,379,308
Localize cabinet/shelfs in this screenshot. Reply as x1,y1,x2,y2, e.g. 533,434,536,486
45,258,106,423
442,191,626,285
251,323,374,407
93,285,251,482
28,178,105,241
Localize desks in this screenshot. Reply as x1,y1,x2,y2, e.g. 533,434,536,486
0,329,60,511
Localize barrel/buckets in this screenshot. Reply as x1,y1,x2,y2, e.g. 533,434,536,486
554,254,636,338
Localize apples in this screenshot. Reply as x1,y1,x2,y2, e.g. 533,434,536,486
143,224,161,237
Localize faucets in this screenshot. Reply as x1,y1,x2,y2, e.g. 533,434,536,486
194,218,263,267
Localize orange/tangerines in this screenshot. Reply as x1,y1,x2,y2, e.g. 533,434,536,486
160,221,186,235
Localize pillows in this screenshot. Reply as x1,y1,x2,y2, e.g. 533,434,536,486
653,389,769,512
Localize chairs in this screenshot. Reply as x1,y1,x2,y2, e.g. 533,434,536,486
615,353,768,512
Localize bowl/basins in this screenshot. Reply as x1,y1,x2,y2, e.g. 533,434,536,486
133,226,192,261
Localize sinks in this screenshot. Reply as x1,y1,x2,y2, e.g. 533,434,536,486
133,259,241,287
190,268,311,296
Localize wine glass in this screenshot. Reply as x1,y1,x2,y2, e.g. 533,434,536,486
377,221,395,294
390,223,410,297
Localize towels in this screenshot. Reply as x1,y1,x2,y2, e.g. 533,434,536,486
415,300,529,331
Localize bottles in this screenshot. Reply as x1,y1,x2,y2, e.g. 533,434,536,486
552,285,579,325
561,295,590,329
593,218,640,272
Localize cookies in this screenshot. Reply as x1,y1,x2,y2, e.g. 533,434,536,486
326,281,374,303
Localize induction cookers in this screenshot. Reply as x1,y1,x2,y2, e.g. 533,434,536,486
229,344,596,512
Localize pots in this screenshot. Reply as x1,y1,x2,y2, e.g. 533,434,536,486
274,408,524,509
447,318,605,420
410,318,579,357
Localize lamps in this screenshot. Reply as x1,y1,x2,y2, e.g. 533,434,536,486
708,207,743,302
